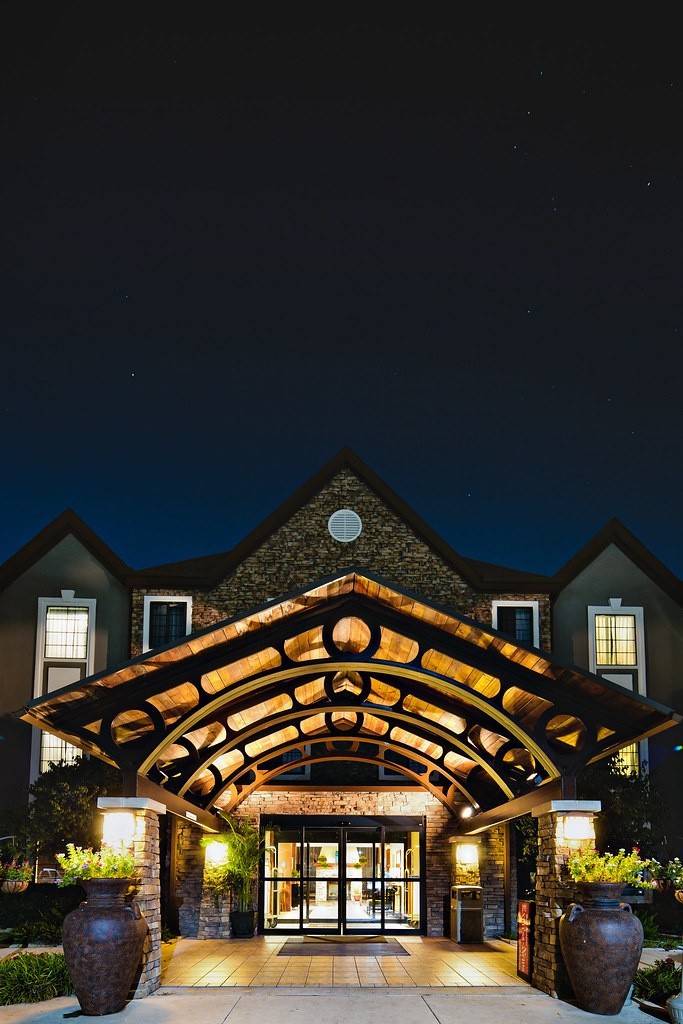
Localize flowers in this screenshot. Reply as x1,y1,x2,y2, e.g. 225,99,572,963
0,857,35,881
54,838,137,888
567,840,658,890
665,857,683,890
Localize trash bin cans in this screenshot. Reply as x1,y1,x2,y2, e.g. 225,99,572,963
451,885,484,945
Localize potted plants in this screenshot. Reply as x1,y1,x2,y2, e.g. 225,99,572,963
317,855,327,865
292,871,299,878
198,810,270,939
353,888,361,901
359,856,368,866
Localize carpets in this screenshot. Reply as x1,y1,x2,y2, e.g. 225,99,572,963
277,937,411,957
302,935,388,943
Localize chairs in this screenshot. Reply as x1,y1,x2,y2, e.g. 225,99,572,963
368,888,394,916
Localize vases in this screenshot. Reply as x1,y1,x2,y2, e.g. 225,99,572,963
0,879,29,893
559,881,644,1016
674,890,683,903
61,879,148,1016
666,969,683,1024
